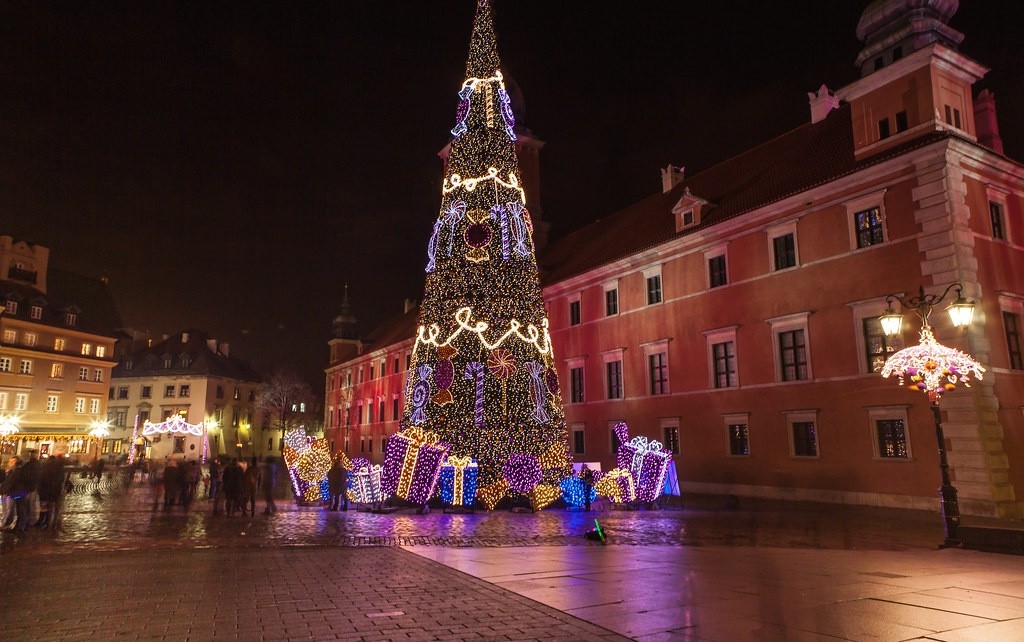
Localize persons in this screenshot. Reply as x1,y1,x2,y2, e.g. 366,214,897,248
97,458,105,482
264,457,276,514
326,457,349,512
161,454,260,517
0,455,66,532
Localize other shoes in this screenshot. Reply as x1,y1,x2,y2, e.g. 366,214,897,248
262,508,269,513
272,506,277,512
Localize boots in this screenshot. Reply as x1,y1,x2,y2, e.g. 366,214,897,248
339,501,347,511
331,501,338,511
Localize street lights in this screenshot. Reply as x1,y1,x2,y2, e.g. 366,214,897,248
877,283,986,550
